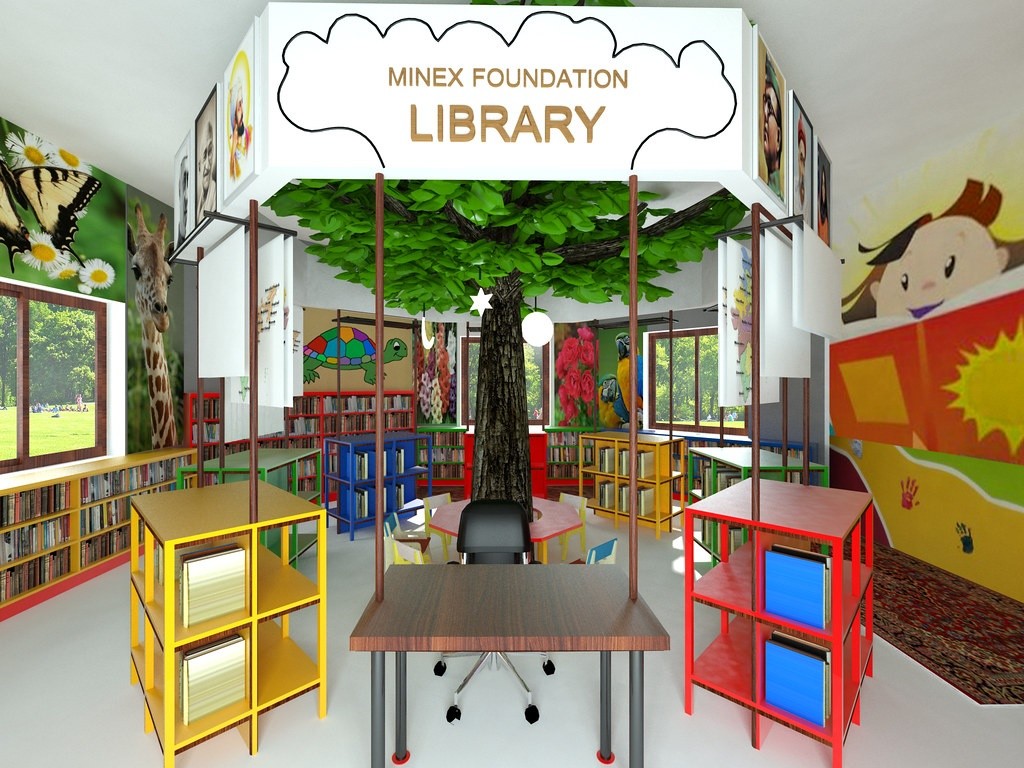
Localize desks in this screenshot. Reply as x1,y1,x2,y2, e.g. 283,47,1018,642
347,563,671,768
428,495,584,566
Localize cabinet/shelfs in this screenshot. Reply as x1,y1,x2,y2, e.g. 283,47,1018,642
0,389,882,768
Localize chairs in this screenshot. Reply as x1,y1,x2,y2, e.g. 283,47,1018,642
391,537,425,565
422,490,455,558
382,510,434,559
559,492,587,562
567,537,618,565
434,497,555,727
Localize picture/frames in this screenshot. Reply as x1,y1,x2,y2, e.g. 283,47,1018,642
751,23,833,249
173,14,261,252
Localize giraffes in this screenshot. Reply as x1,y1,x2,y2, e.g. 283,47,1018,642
128,203,179,449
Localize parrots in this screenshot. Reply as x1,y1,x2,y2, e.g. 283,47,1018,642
599,332,644,429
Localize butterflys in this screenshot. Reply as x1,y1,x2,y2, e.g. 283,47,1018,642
0,144,102,274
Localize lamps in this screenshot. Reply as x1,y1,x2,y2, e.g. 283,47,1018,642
521,296,554,348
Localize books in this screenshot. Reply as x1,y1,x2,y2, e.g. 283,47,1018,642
0,398,247,725
545,429,833,728
260,394,465,520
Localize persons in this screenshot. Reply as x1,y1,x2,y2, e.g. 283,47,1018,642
707,411,739,421
533,409,539,419
0,394,89,418
